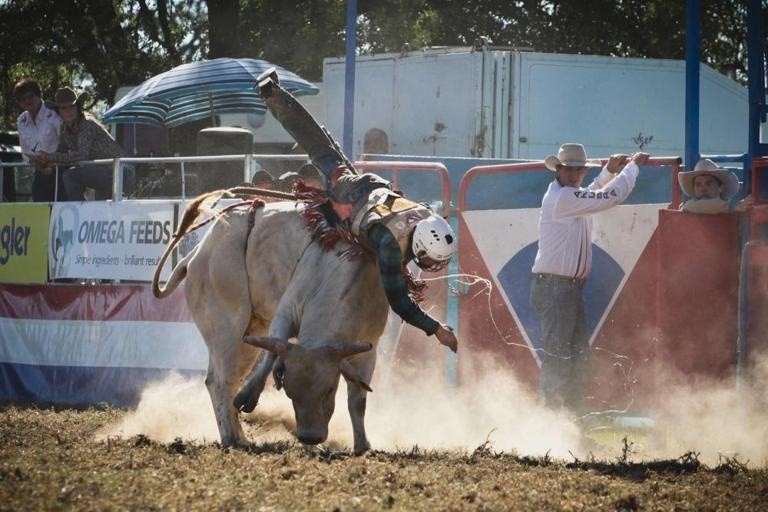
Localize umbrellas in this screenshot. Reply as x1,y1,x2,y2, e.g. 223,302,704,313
98,56,322,128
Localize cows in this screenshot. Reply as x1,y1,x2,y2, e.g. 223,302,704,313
152,189,390,457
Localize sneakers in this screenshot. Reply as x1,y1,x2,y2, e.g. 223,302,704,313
256,65,278,97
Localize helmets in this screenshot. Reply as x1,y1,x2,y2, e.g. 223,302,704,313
412,214,457,272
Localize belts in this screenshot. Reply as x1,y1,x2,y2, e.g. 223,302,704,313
539,273,585,285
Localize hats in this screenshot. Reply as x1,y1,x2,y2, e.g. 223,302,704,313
543,143,602,171
677,157,739,198
253,161,321,184
44,87,89,109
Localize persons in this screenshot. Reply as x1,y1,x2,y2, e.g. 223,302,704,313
126,127,391,200
251,67,458,354
676,158,754,215
13,79,66,201
28,87,134,201
529,143,650,420
